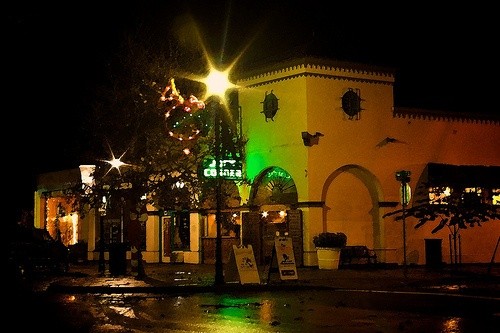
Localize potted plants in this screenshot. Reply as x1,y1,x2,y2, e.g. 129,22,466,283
313,232,348,271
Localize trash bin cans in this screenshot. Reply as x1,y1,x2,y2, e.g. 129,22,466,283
424,238,443,270
109,241,126,276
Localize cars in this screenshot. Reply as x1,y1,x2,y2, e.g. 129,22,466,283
6,228,72,278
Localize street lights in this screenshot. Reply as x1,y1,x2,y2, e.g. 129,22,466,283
210,66,227,286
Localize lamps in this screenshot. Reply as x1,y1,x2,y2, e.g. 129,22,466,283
56,202,66,217
140,193,147,205
99,195,107,216
172,179,184,193
